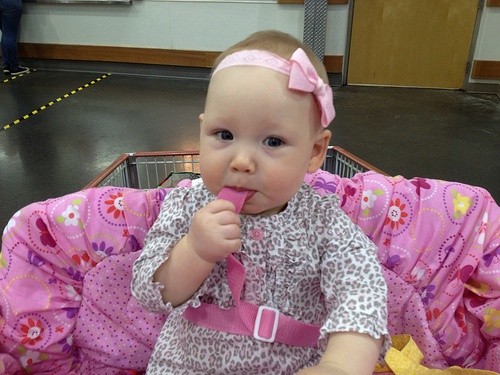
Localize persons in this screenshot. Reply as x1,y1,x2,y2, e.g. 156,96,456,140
129,29,393,374
